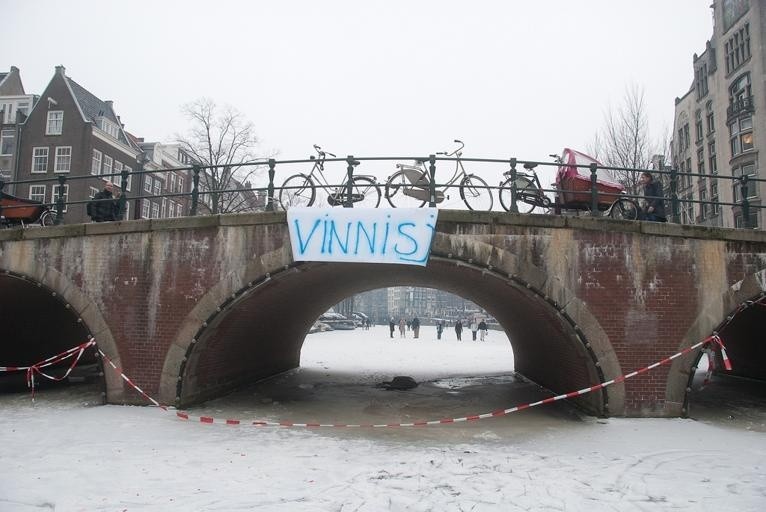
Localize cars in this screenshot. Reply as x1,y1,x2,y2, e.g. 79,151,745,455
308,312,368,333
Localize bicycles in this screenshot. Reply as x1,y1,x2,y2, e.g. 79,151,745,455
498,148,639,221
40,193,57,227
278,144,382,211
384,139,494,212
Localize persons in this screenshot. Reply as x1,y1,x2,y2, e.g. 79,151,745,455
90,181,116,222
477,318,488,341
437,323,443,340
625,199,644,221
641,170,667,223
455,319,463,341
360,317,376,330
389,318,395,338
398,316,420,338
470,319,479,341
111,189,130,221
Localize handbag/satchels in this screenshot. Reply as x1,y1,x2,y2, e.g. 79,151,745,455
87,198,96,215
